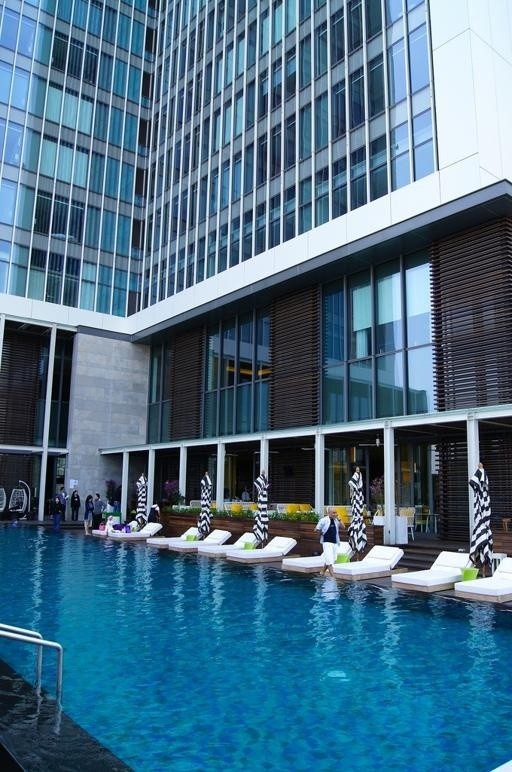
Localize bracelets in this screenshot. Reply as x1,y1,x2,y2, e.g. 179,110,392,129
338,519,341,522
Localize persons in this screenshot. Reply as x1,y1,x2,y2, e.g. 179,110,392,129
313,507,346,576
148,501,162,522
51,486,107,537
12,518,18,527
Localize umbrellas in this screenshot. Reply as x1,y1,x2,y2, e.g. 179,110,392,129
196,471,213,539
469,462,494,577
135,472,148,525
252,470,271,549
347,466,367,562
